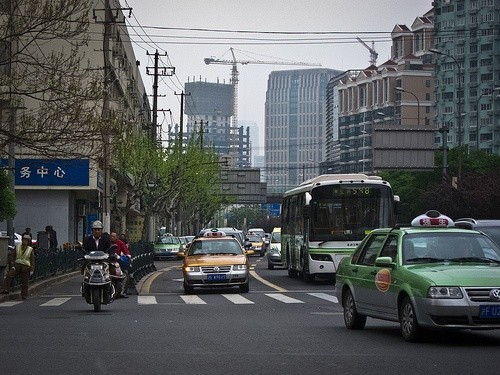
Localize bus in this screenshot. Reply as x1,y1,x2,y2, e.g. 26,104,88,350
279,172,402,283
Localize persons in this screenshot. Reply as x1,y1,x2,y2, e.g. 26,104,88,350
109,233,133,297
80,220,116,288
45,226,58,249
119,234,128,244
0,235,35,300
22,228,32,246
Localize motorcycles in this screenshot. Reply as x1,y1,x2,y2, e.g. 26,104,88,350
75,239,132,312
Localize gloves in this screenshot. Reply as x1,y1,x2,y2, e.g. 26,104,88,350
11,267,16,271
30,271,33,276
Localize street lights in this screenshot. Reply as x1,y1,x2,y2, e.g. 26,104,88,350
476,86,500,153
448,116,465,150
394,86,420,126
428,47,462,146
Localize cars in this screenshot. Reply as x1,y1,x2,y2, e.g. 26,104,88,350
452,217,500,259
335,210,500,342
176,230,255,296
152,226,281,270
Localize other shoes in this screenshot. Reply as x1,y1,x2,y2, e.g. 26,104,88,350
22,295,27,299
0,289,9,294
118,295,128,298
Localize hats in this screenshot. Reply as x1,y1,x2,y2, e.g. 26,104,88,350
23,235,32,239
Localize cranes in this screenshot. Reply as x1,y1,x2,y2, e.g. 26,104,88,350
355,35,379,68
202,46,324,169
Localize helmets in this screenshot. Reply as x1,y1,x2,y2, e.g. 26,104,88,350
92,220,103,228
